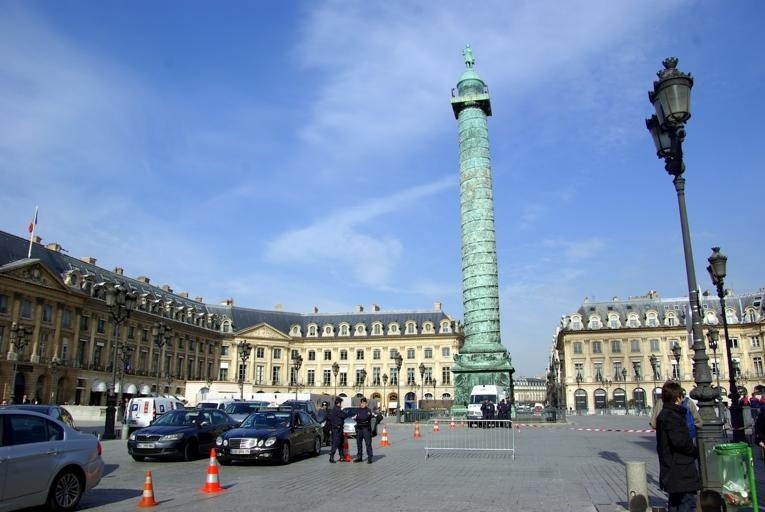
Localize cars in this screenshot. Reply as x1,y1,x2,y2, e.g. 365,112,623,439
129,407,242,464
225,400,272,427
314,394,342,424
338,405,378,438
0,403,73,436
215,413,325,465
0,409,104,512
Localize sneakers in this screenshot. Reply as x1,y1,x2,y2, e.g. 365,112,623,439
341,456,345,461
330,458,336,463
353,456,362,462
367,459,372,464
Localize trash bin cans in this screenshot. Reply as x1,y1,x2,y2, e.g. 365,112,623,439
712,441,759,512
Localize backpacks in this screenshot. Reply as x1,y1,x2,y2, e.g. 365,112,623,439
680,398,696,438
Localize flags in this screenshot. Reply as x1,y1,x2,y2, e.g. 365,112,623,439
28,213,37,232
124,361,132,373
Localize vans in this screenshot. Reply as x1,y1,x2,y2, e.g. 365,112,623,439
467,383,509,428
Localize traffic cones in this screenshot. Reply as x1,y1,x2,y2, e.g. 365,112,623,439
198,448,225,494
412,421,422,439
449,416,457,430
138,471,159,507
458,415,465,429
380,425,392,448
338,432,354,463
433,417,441,432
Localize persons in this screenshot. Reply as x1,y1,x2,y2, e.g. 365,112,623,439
351,398,373,464
481,397,513,428
318,401,332,446
649,380,765,512
329,398,348,463
2,394,129,411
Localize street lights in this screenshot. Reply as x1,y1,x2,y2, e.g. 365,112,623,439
707,244,750,447
575,374,584,417
8,321,34,403
645,57,729,512
649,354,660,390
118,339,136,403
330,360,341,395
622,368,629,414
707,325,724,398
634,361,642,415
47,356,63,405
671,341,683,384
93,277,139,441
237,339,256,401
292,353,305,399
358,351,436,423
602,378,612,413
149,320,173,395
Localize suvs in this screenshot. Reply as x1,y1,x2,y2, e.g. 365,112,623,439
124,395,188,429
277,400,315,414
197,399,234,411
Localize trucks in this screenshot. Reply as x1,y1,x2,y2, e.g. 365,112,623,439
58,403,124,443
194,393,314,412
